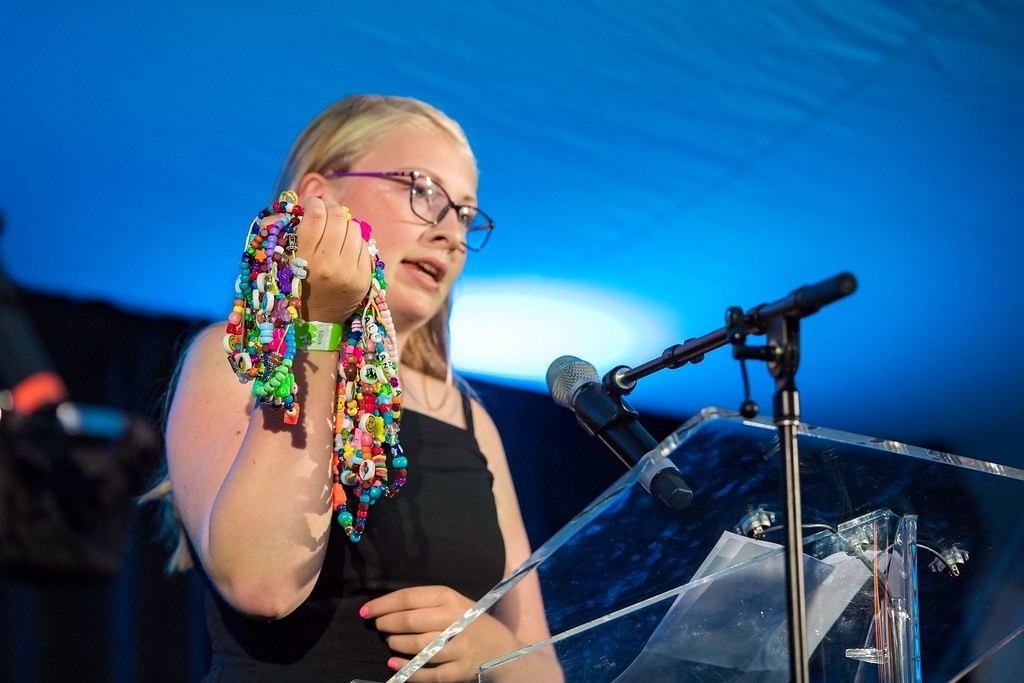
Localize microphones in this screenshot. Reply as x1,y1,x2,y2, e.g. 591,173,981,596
546,356,693,511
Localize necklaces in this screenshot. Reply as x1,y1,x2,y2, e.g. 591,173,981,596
223,193,407,541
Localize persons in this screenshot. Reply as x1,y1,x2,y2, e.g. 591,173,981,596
140,96,564,683
0,267,67,683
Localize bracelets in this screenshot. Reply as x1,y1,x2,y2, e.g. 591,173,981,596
293,319,343,353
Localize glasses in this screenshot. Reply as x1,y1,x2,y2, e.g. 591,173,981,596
325,169,495,252
